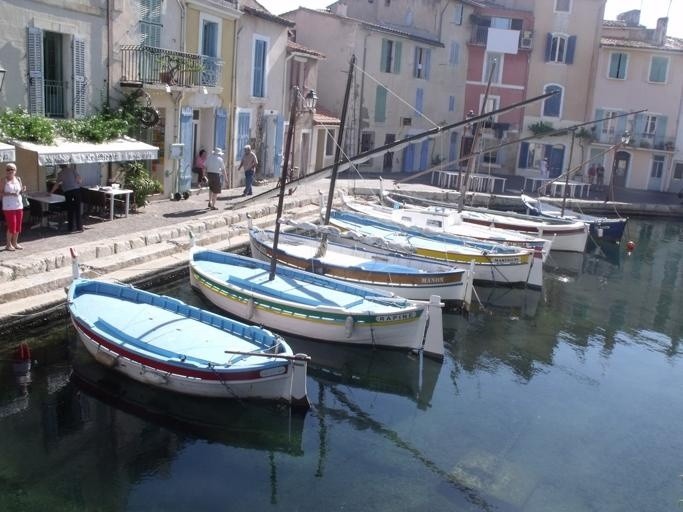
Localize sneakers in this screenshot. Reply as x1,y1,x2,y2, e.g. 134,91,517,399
208,204,211,207
211,207,218,210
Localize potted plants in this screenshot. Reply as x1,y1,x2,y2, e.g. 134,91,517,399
155,56,174,83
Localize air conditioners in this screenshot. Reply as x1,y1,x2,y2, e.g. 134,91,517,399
520,38,532,49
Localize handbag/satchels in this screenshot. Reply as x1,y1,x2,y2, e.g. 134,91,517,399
20,192,30,208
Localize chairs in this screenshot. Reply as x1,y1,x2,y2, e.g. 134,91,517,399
79,182,138,215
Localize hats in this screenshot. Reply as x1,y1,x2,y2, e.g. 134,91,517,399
212,148,224,156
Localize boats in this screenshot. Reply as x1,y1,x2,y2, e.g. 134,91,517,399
64,272,305,406
330,197,629,290
246,223,472,309
181,240,449,359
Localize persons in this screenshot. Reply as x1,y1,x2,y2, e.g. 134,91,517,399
13,343,35,401
238,144,259,197
587,164,596,191
539,157,549,177
192,148,209,187
203,147,230,211
0,161,29,251
595,163,605,192
45,164,84,232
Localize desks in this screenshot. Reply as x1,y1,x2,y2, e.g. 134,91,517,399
24,190,65,231
83,184,133,220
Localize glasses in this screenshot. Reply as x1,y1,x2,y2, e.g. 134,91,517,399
7,169,15,172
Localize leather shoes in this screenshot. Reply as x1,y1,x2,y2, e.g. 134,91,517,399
12,243,24,249
6,245,16,251
